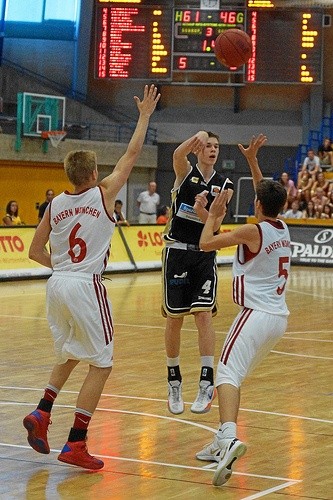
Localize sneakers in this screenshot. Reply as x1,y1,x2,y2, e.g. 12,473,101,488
167,377,184,414
195,442,221,462
57,440,104,470
190,380,218,413
23,408,52,454
213,439,247,486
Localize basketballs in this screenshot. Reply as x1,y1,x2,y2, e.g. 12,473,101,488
215,28,252,68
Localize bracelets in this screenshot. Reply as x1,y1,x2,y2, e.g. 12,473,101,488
298,189,302,193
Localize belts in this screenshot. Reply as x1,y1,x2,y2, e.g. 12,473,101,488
140,212,156,215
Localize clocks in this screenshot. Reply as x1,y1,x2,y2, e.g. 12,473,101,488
200,0,220,10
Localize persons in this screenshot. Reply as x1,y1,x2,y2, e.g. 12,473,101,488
38,189,55,225
284,201,302,219
312,187,328,210
301,149,322,182
158,206,170,223
23,83,161,468
328,183,333,203
297,169,313,201
313,173,329,197
303,200,319,219
320,204,333,220
317,138,333,172
277,172,297,214
112,200,129,227
2,200,26,226
161,130,234,415
136,181,160,223
195,133,292,486
